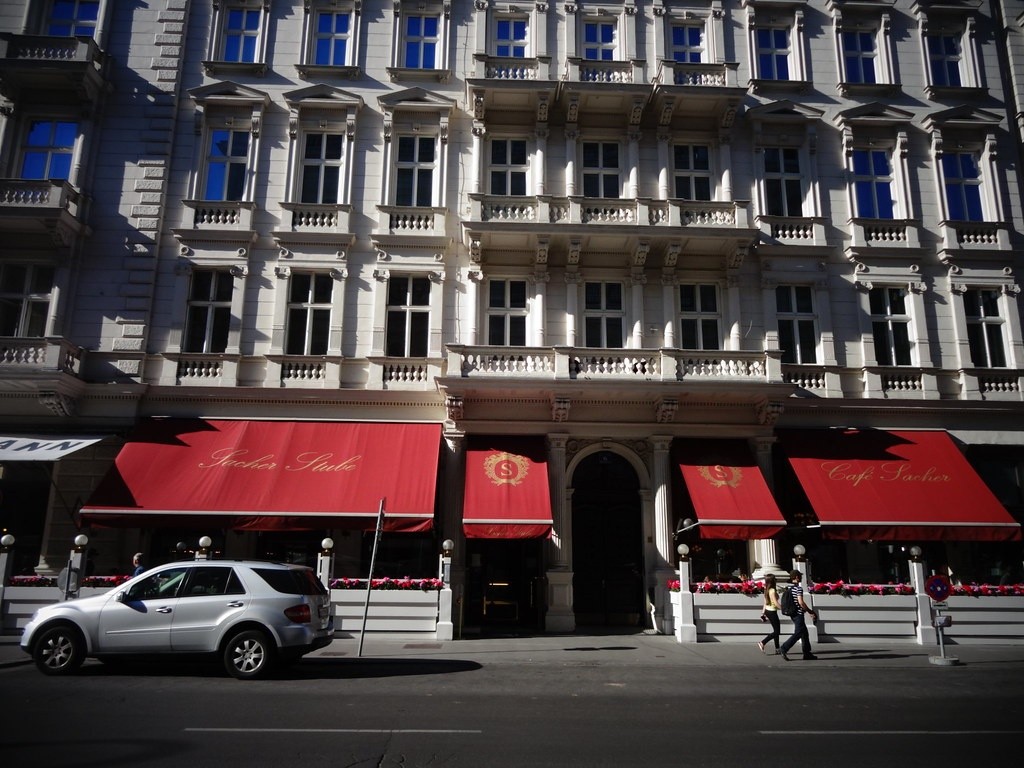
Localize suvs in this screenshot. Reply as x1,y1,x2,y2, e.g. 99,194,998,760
19,558,336,680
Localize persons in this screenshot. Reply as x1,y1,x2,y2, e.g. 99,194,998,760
999,563,1024,587
131,552,145,594
757,574,782,655
780,569,818,661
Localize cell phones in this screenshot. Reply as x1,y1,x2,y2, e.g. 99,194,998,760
761,616,768,622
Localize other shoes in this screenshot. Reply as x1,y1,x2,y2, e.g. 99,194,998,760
775,649,780,654
778,650,789,661
758,642,764,652
803,652,820,660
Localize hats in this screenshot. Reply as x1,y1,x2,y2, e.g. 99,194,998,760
790,570,803,578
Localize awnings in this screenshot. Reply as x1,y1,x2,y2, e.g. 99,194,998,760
461,431,553,541
671,434,787,542
77,415,442,536
1,431,119,463
785,428,1024,545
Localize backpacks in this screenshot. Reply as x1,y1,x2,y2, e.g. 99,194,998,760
781,585,802,616
